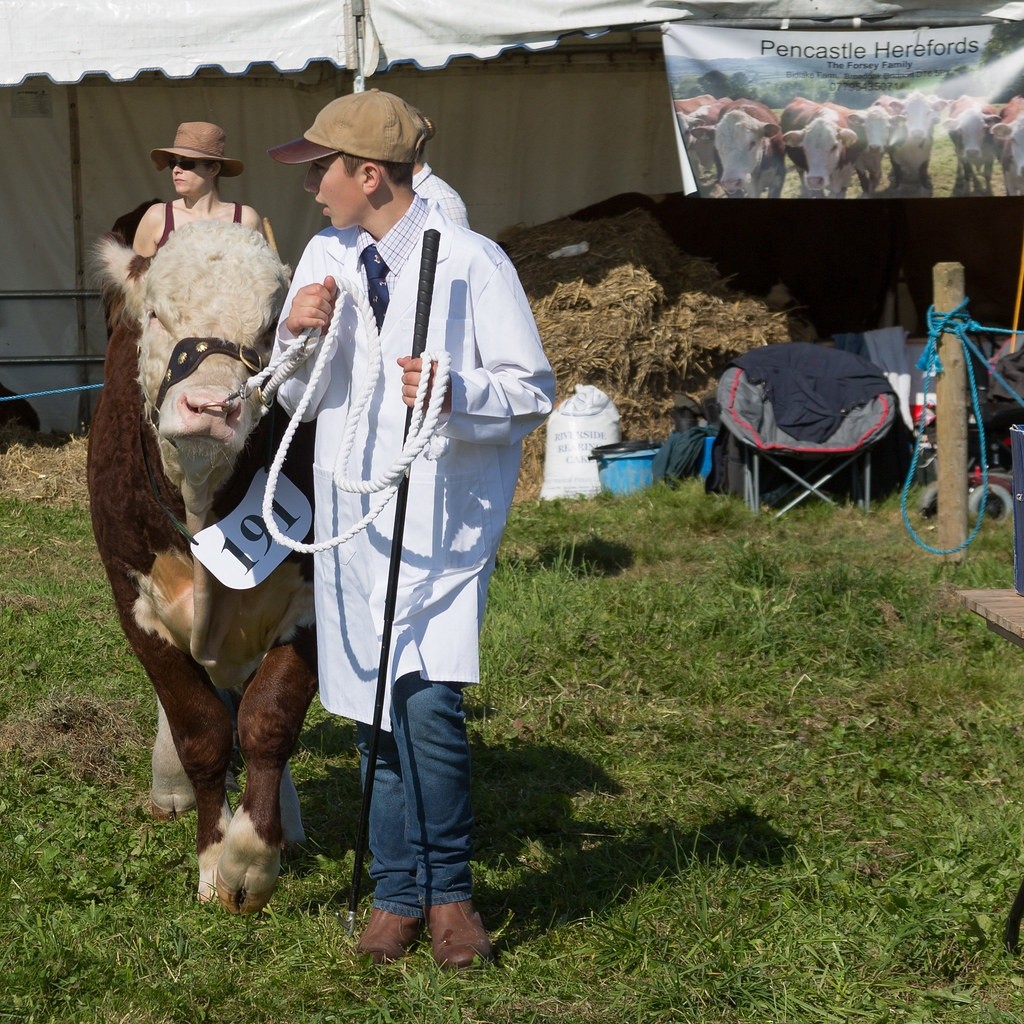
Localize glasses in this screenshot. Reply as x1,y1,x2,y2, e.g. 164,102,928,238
168,157,208,170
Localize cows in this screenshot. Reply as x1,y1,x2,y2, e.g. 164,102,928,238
81,224,320,921
674,87,1024,197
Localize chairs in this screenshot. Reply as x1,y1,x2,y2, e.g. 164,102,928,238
714,343,902,522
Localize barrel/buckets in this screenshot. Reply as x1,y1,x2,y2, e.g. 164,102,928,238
1009,425,1024,597
587,441,662,499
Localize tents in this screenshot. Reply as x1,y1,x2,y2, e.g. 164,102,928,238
0,0,1024,434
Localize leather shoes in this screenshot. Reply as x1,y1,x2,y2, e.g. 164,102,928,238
423,898,490,968
357,908,426,964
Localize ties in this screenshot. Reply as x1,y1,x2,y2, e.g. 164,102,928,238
361,246,390,336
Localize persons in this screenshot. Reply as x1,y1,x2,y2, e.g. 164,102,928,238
133,121,265,262
266,88,554,973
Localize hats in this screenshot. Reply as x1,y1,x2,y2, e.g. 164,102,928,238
150,122,244,177
267,89,424,165
